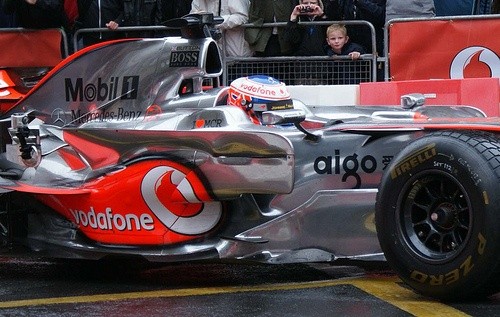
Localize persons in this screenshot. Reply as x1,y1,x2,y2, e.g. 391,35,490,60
190,0,252,59
248,0,293,80
285,0,331,85
227,74,296,132
386,0,500,28
1,0,187,54
340,0,386,82
326,24,366,84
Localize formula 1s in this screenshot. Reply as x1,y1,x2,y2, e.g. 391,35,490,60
0,11,500,296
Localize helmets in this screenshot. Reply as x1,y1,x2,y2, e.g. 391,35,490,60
226,74,293,125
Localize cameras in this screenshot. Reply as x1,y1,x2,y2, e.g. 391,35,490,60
210,27,224,42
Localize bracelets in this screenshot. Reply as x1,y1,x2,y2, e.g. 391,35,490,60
319,13,326,18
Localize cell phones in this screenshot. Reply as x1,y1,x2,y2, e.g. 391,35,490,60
299,5,313,13
